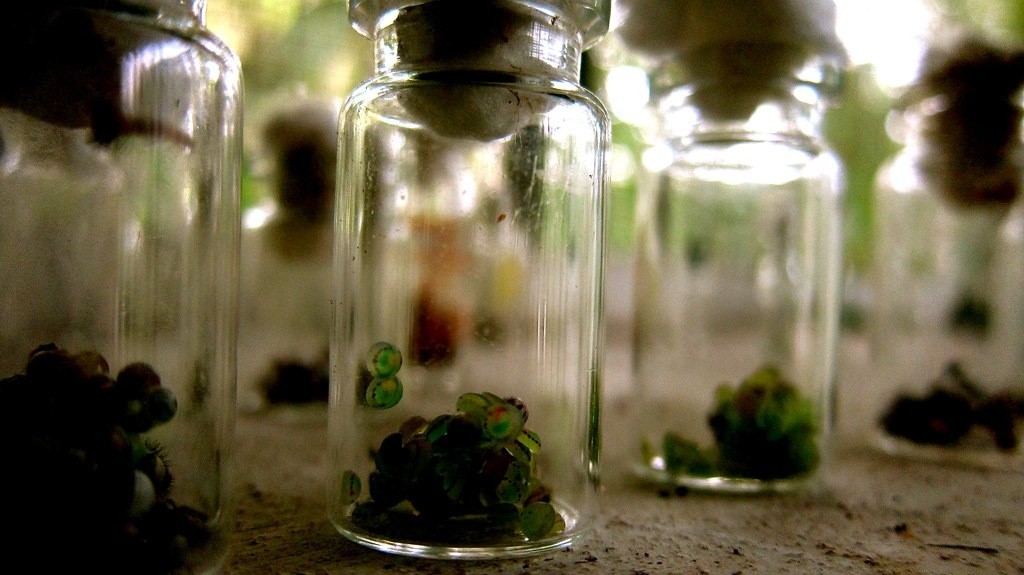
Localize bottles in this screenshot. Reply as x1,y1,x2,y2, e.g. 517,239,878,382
627,40,840,496
1,0,243,574
325,1,611,560
840,40,1024,472
